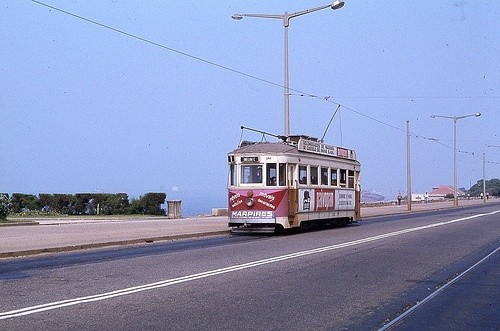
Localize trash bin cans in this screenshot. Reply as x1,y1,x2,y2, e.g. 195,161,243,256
167,200,183,219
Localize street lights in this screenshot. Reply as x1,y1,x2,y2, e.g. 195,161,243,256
231,0,347,140
430,112,481,207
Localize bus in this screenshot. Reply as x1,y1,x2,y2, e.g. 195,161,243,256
227,106,362,234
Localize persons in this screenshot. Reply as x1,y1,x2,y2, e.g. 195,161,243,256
481,193,483,199
487,193,489,199
425,191,428,204
397,193,402,205
270,175,327,185
467,194,470,200
